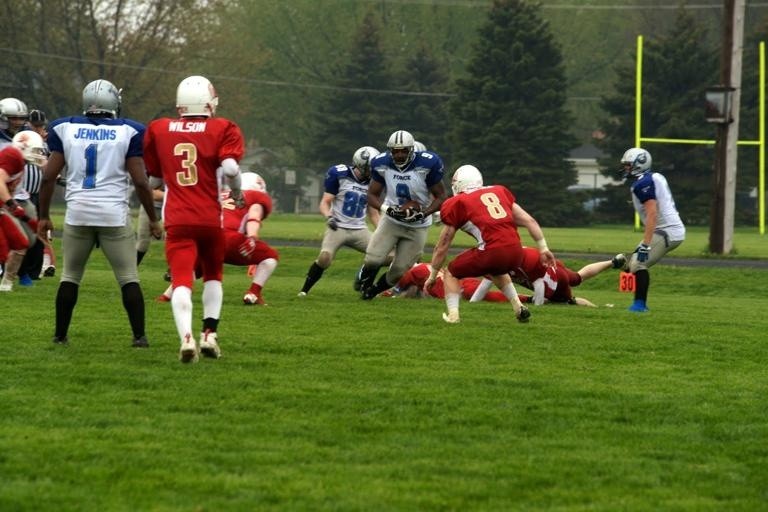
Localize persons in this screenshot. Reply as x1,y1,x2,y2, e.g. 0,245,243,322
1,96,26,145
623,149,686,310
7,132,52,286
132,173,176,281
355,131,449,299
426,164,555,323
39,80,161,348
141,76,249,363
26,108,48,137
468,247,632,308
153,169,277,305
388,263,544,303
297,146,380,296
1,131,54,293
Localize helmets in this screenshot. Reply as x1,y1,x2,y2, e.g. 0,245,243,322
241,172,267,194
0,97,31,121
450,164,484,195
352,145,381,182
414,139,427,153
80,78,123,120
386,129,415,168
11,129,49,167
619,147,653,178
175,76,219,117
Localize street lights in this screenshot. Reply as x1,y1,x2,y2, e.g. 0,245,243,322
706,83,734,253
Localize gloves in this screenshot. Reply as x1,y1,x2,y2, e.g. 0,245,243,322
326,216,342,231
149,221,162,241
28,218,38,233
386,205,414,221
35,217,56,249
401,206,425,223
229,190,248,211
6,197,25,218
633,244,651,262
238,236,259,256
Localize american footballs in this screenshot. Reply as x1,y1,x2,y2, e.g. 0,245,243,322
400,200,420,217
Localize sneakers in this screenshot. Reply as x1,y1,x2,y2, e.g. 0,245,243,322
242,291,269,309
629,300,649,313
52,333,69,346
353,265,368,292
441,312,461,325
516,305,532,323
298,288,312,298
42,264,57,278
197,330,224,361
360,280,377,301
18,272,33,287
157,293,172,304
178,336,200,365
0,277,15,292
162,269,174,283
130,335,149,348
612,253,631,273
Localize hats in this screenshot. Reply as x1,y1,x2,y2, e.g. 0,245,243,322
30,109,49,126
